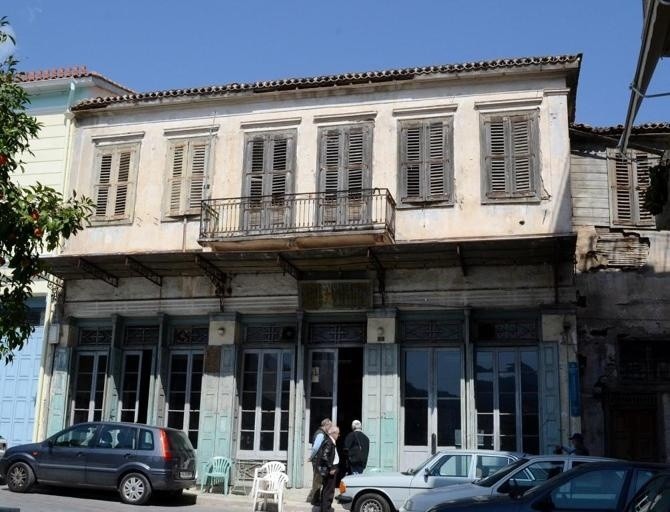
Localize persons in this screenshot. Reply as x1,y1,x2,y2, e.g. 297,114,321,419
317,426,340,512
307,418,333,502
568,433,589,455
344,419,370,475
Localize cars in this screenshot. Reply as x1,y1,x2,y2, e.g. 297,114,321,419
421,455,670,512
398,452,653,512
0,419,199,506
334,446,554,512
623,471,670,512
0,435,8,461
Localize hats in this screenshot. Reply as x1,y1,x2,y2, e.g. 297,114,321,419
569,433,584,440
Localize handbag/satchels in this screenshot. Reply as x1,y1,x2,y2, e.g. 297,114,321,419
347,432,362,466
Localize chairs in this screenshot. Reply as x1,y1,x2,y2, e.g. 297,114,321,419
114,432,126,448
249,461,284,498
97,431,113,448
252,471,290,512
203,457,232,496
